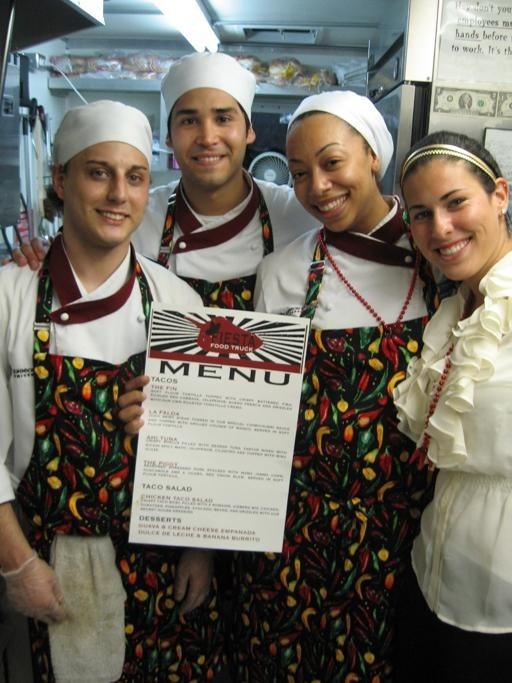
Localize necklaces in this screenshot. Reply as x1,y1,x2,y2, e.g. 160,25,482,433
315,230,423,375
407,293,478,470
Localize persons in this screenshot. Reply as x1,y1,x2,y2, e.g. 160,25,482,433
115,89,457,681
1,96,234,681
390,126,511,682
0,47,409,680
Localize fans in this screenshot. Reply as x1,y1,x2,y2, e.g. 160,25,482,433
247,151,294,191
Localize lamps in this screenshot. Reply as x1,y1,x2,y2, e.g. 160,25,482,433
147,0,221,53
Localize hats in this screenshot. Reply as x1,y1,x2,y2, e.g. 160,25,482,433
161,51,255,123
288,91,394,182
51,100,153,172
